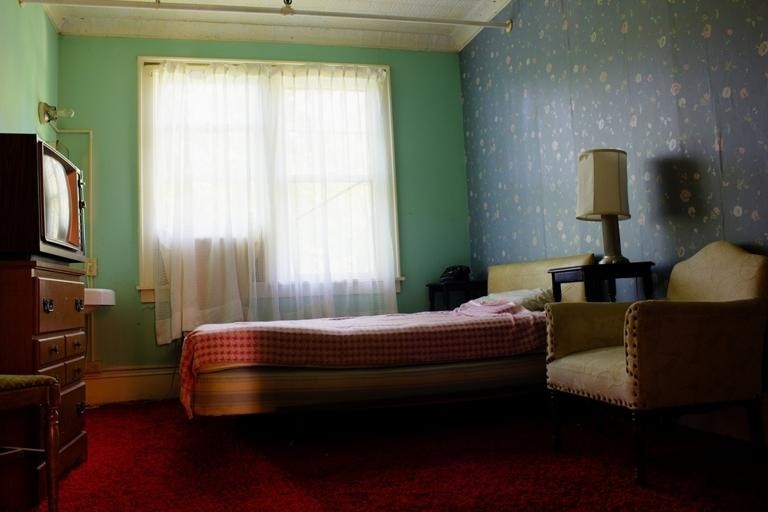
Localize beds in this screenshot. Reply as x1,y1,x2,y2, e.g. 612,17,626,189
177,251,597,421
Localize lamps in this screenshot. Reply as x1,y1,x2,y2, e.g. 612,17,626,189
573,146,634,266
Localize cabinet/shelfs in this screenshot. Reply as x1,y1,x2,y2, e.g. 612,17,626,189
0,261,89,510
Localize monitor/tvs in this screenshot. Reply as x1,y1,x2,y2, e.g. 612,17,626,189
0,133,86,266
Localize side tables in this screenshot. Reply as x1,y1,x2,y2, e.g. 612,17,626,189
423,281,488,311
546,259,656,306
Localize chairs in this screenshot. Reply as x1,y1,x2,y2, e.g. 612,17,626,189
540,240,766,491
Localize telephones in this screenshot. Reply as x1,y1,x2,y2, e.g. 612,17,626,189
440,265,470,284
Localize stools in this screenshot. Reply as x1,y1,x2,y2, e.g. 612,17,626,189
0,372,66,510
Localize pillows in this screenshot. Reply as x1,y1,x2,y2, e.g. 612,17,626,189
481,286,547,310
